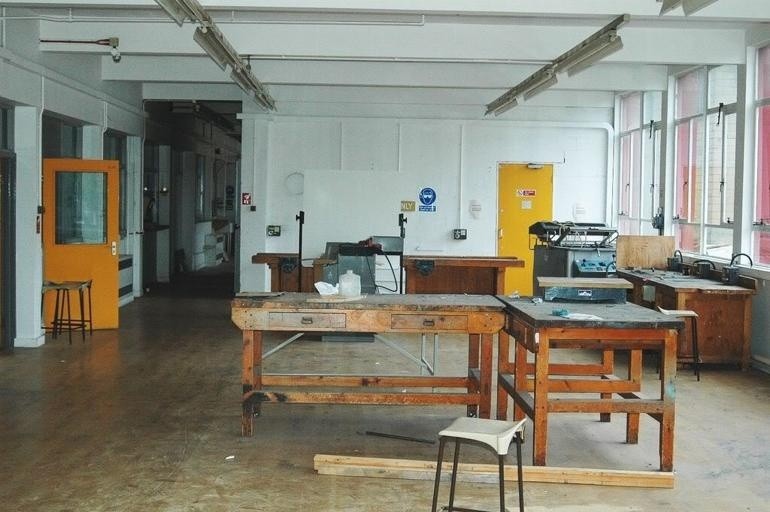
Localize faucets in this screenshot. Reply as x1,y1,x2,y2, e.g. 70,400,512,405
730,253,753,267
674,250,683,263
692,259,716,269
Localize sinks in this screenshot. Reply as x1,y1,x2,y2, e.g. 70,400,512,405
640,269,700,281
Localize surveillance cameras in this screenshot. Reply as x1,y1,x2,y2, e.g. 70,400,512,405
111,47,121,63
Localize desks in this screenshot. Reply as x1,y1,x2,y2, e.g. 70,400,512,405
496,295,686,472
230,290,507,436
400,256,525,295
617,264,758,373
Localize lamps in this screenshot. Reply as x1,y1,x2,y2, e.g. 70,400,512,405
156,0,279,116
483,0,717,120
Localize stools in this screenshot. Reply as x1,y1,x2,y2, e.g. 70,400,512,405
656,306,700,381
431,417,528,511
40,280,93,345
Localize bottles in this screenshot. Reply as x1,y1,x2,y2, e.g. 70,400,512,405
339,270,361,298
